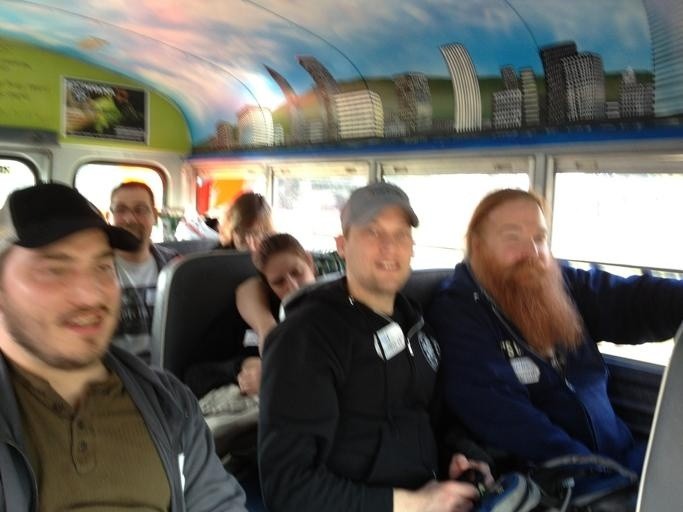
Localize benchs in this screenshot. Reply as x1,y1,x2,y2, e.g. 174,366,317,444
146,239,682,512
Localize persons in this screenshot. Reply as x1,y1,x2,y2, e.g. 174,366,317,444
215,191,317,397
256,182,498,512
1,178,250,512
426,187,683,512
108,181,182,369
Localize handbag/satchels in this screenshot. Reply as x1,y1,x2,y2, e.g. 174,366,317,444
468,469,540,512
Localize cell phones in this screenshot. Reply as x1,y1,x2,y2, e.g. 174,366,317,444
455,467,483,489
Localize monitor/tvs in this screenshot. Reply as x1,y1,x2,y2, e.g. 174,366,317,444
60,76,150,142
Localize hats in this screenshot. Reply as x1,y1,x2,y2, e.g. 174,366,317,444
0,182,142,260
340,182,421,241
